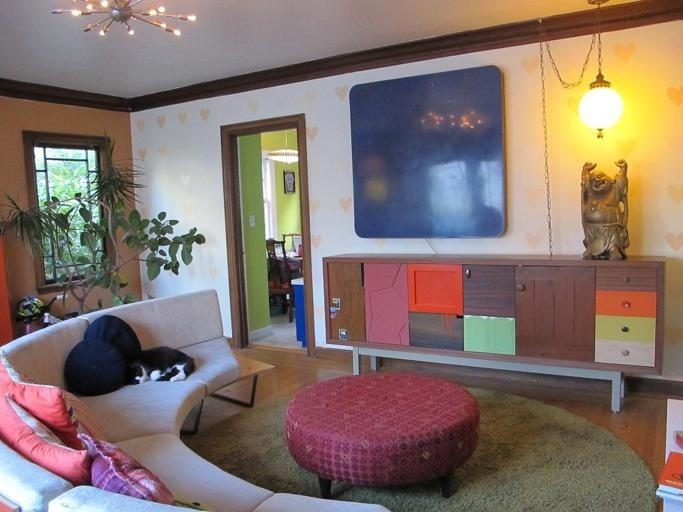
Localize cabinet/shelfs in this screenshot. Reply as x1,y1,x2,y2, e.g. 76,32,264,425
322,254,667,413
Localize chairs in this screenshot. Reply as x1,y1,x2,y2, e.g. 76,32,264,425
265,233,304,322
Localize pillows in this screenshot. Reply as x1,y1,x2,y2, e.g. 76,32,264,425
0,348,174,505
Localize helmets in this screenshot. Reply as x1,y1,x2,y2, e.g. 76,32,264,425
15,297,46,322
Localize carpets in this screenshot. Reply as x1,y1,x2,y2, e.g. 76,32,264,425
182,387,657,512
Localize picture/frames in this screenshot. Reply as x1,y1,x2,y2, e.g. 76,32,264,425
283,170,295,194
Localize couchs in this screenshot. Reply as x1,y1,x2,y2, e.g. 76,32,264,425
0,289,391,512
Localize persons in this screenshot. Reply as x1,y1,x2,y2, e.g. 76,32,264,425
579,156,631,260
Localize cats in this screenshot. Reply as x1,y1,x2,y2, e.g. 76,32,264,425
127,346,195,384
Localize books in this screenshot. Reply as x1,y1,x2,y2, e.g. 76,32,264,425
653,427,683,505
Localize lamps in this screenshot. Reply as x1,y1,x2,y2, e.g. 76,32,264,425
51,0,197,36
266,132,298,166
579,0,622,138
